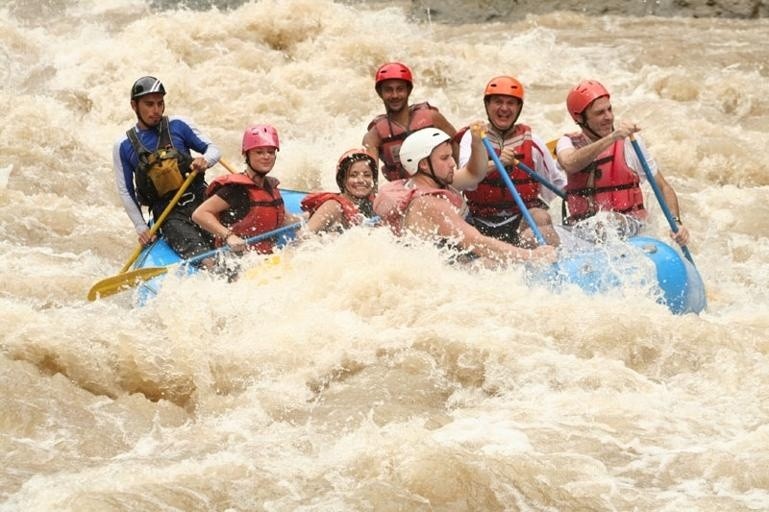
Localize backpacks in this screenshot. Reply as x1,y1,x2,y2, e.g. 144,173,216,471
125,115,204,214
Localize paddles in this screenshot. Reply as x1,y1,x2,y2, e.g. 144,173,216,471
87,220,311,300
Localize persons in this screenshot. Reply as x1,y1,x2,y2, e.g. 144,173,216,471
190,125,299,264
450,75,561,265
358,61,461,184
297,148,379,240
384,126,558,267
553,77,692,250
111,76,222,278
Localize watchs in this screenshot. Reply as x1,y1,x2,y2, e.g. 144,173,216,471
670,215,681,223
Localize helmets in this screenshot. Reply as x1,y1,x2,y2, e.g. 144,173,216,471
375,62,412,93
566,79,610,121
130,76,164,99
241,124,279,155
398,127,451,177
484,75,523,104
335,148,378,186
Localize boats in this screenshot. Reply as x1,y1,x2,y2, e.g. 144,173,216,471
133,189,704,320
